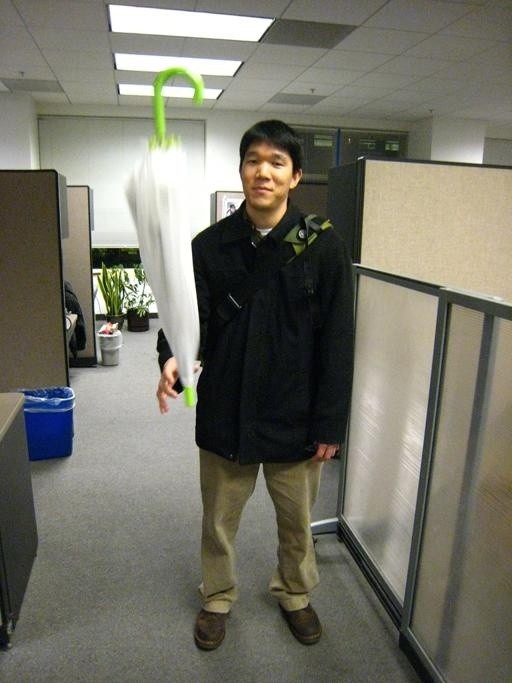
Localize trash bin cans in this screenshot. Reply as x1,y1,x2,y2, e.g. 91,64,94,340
17,387,76,461
96,330,121,366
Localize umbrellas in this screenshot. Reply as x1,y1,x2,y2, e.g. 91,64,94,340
120,60,218,408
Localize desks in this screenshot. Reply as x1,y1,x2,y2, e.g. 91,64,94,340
64,310,79,372
0,388,40,651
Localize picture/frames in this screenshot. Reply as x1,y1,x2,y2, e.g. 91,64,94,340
212,187,247,224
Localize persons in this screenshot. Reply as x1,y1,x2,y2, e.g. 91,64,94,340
154,117,355,652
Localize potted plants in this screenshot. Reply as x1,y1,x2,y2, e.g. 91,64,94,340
122,261,157,333
95,258,133,331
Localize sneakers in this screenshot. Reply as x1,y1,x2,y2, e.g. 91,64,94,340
278,599,323,646
193,608,231,651
